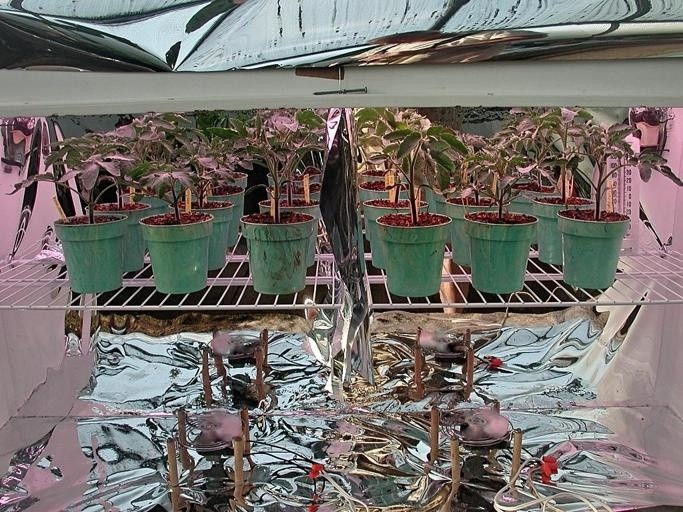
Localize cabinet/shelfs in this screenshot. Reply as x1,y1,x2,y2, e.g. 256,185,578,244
1,58,683,324
